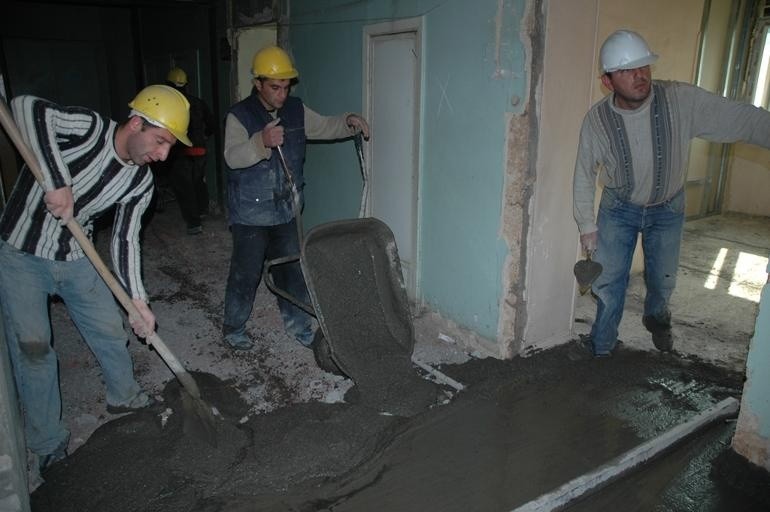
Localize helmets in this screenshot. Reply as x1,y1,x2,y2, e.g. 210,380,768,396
254,46,299,81
599,28,659,74
128,83,193,147
166,68,186,86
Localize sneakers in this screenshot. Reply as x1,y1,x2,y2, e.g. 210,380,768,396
227,330,253,350
295,328,315,346
187,225,203,235
593,347,610,361
107,394,159,414
38,449,68,483
652,328,673,353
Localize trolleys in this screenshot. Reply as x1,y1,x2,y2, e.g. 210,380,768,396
262,125,417,386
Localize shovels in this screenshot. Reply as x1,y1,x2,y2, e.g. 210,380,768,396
0,101,225,445
574,251,603,296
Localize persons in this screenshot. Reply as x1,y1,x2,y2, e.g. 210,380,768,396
570,28,770,361
219,45,370,352
0,85,195,481
165,67,215,234
